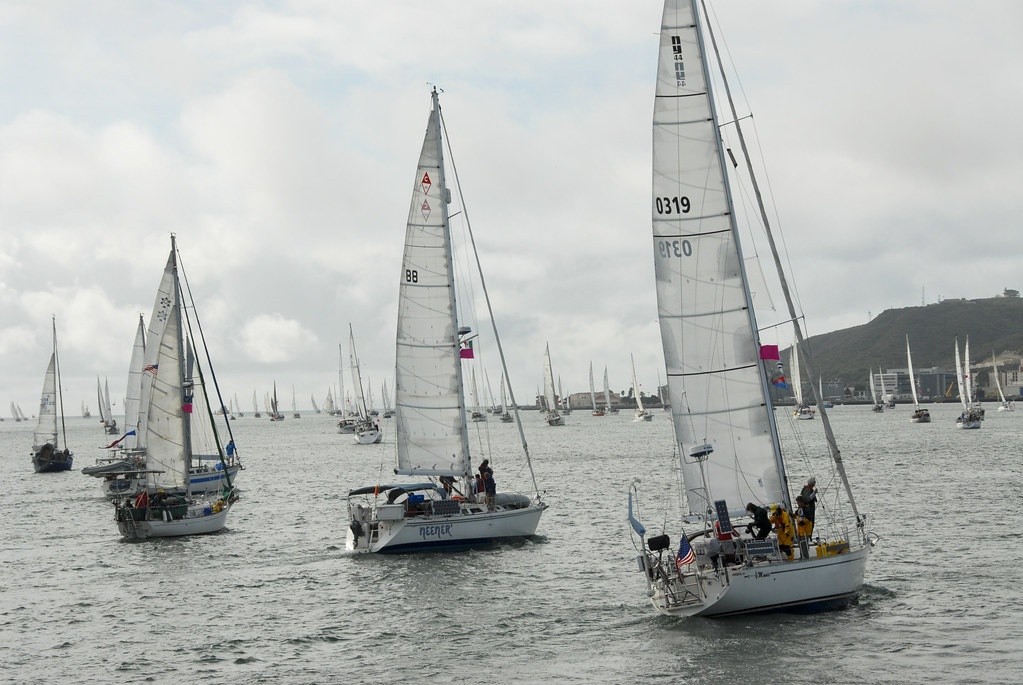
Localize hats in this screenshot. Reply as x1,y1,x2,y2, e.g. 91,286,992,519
807,477,815,484
770,503,778,513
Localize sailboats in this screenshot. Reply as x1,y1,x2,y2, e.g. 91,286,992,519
536,341,574,428
499,370,515,424
788,333,816,420
96,375,121,435
817,370,834,409
466,365,504,423
10,401,27,422
81,229,247,541
341,82,551,557
904,333,931,424
878,364,896,409
869,366,884,412
656,367,672,413
588,360,605,416
29,311,74,474
252,322,397,447
629,353,655,421
624,0,883,622
954,334,987,429
601,365,621,416
991,348,1016,412
81,399,92,419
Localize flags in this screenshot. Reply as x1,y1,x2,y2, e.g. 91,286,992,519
97,430,137,449
772,376,787,390
460,348,473,358
676,533,696,569
760,345,779,360
181,404,193,413
142,364,158,375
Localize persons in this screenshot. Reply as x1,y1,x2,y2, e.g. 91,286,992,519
473,474,485,494
714,521,740,564
123,488,168,508
483,473,496,513
440,476,458,497
226,440,238,468
746,478,818,560
478,459,494,504
